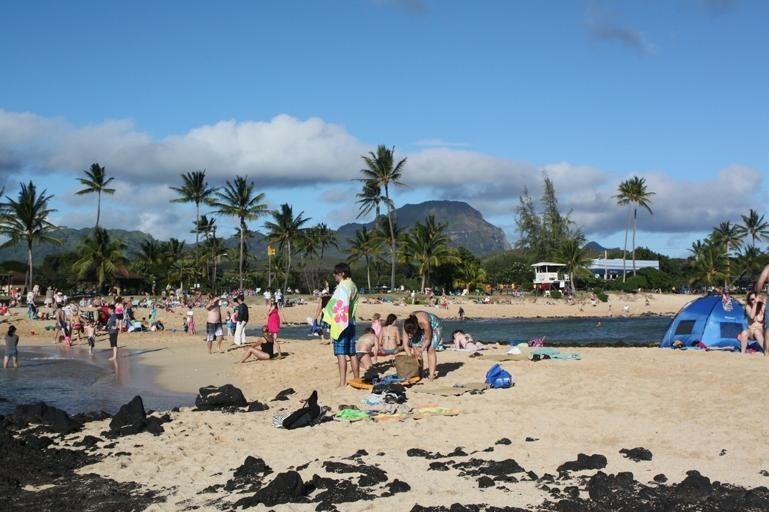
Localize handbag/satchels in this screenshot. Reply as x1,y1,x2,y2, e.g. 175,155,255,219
487,365,511,388
395,355,420,377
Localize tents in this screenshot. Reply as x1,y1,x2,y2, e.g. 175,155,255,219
658,291,764,353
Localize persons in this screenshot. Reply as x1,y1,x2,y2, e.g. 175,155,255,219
371,313,381,355
756,264,768,355
102,304,118,361
458,307,464,320
367,296,382,304
499,284,503,295
478,296,491,304
579,303,583,311
430,296,449,310
624,305,629,313
740,291,764,353
354,328,378,371
451,298,465,304
452,330,483,349
317,262,359,388
4,326,19,368
609,305,613,318
25,284,202,358
205,286,305,365
403,311,444,382
2,284,27,316
645,299,649,305
378,313,402,356
